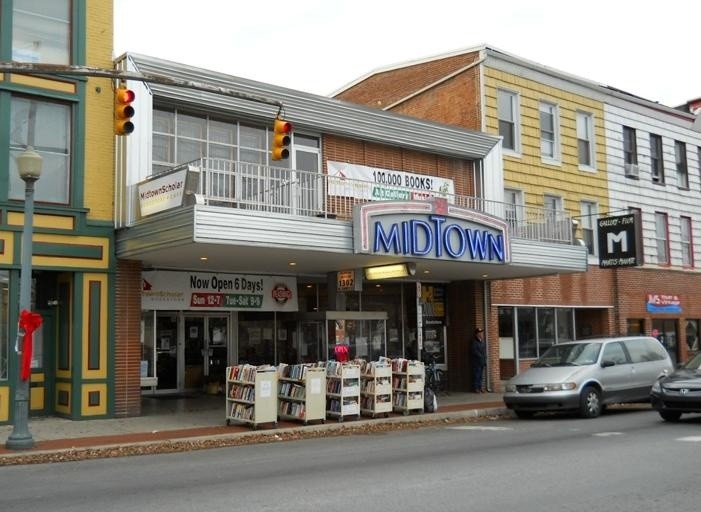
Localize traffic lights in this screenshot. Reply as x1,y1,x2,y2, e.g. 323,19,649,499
113,88,135,136
271,117,292,161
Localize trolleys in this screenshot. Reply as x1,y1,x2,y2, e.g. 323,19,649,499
225,360,426,430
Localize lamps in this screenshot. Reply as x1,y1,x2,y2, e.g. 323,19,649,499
364,261,417,280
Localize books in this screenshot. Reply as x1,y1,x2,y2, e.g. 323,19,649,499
318,359,357,413
349,358,388,410
380,356,421,408
227,363,274,421
278,362,316,419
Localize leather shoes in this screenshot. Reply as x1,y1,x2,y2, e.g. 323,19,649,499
474,388,488,393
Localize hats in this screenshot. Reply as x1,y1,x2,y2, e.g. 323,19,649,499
476,328,484,333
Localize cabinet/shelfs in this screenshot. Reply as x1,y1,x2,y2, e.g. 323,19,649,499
225,355,426,430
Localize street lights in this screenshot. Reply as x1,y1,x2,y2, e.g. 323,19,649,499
5,145,43,451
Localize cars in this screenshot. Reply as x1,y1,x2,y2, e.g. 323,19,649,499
650,349,700,421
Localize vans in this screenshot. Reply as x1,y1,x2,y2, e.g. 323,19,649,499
503,336,674,419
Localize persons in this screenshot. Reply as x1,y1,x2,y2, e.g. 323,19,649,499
470,327,487,393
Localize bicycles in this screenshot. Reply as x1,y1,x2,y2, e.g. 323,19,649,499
425,352,450,397
